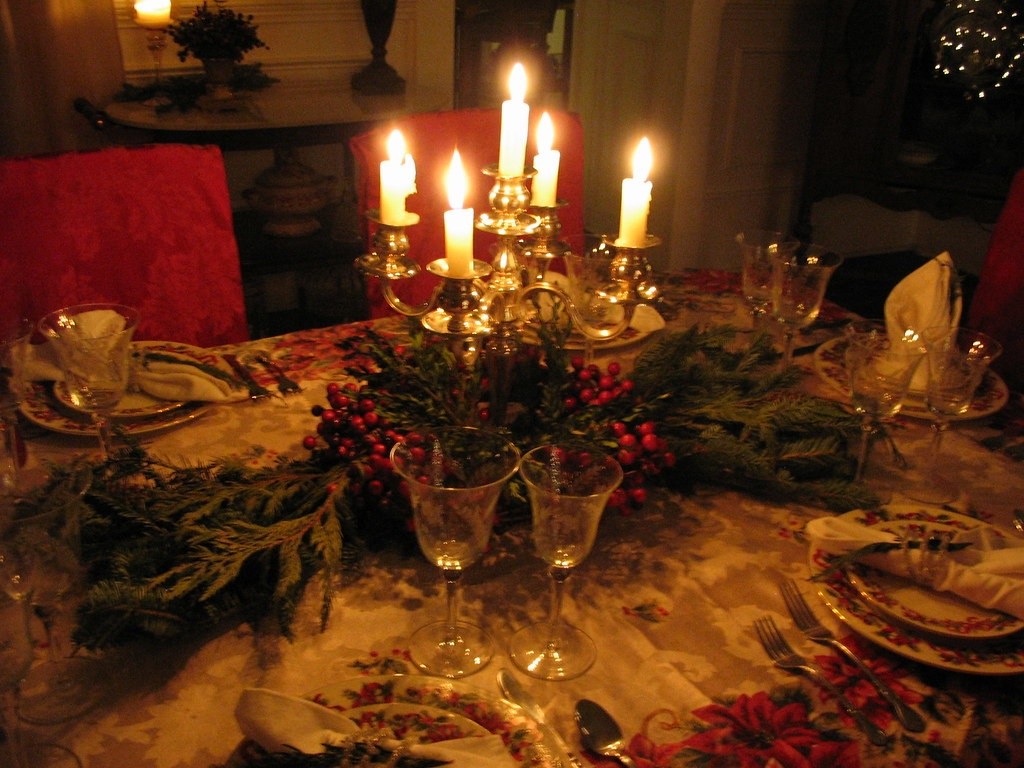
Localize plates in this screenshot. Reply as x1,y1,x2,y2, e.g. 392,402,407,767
812,334,1008,420
299,679,575,768
844,513,1024,639
21,340,221,436
805,512,1023,678
513,303,655,349
53,350,200,418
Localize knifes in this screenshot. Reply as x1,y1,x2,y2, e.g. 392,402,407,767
497,668,581,767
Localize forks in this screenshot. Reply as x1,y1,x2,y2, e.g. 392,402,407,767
755,615,888,744
225,353,278,401
777,579,926,732
255,354,306,395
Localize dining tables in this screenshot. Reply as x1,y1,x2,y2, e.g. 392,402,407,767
0,269,1024,768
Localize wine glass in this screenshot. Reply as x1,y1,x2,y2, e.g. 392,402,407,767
902,323,1002,503
510,444,623,680
739,229,797,346
1,317,33,494
385,427,517,681
767,241,841,361
42,305,138,462
837,320,925,505
0,455,110,723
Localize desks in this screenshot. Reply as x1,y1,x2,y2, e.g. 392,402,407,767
100,76,447,237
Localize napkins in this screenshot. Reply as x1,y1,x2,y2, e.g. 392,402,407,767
11,340,271,402
800,517,1024,621
233,688,519,768
876,249,966,398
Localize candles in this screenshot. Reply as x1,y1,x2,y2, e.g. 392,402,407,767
378,129,417,223
615,136,653,247
443,146,476,279
529,111,562,208
497,62,531,177
133,0,173,25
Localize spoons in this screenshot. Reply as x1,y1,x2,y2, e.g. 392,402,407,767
575,699,637,768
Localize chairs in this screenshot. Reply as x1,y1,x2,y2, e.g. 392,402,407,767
347,106,584,325
0,142,251,380
